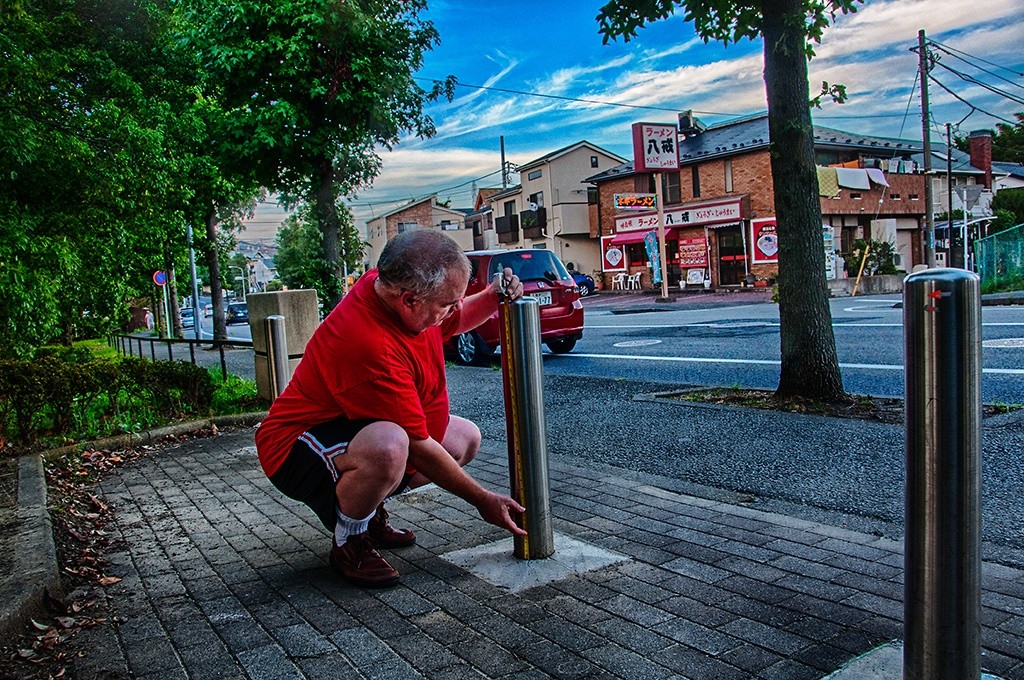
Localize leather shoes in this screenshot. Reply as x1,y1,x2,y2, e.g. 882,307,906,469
368,500,417,549
329,530,400,589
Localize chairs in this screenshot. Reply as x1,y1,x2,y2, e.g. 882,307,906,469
519,264,538,277
612,272,643,290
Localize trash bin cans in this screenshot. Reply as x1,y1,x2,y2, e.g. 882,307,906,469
936,252,946,268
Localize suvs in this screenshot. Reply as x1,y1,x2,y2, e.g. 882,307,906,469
442,248,585,366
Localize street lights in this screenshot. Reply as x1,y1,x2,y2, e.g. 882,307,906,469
228,265,246,302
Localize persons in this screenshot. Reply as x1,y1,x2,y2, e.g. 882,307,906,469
254,229,528,587
144,310,154,330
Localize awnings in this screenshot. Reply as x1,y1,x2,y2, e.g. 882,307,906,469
611,228,672,246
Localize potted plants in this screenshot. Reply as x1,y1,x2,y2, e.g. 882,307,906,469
747,273,755,283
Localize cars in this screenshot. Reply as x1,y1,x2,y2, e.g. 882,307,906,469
224,301,251,325
179,306,195,329
204,303,213,318
567,269,596,298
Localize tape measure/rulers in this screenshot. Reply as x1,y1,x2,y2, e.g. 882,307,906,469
494,273,530,562
